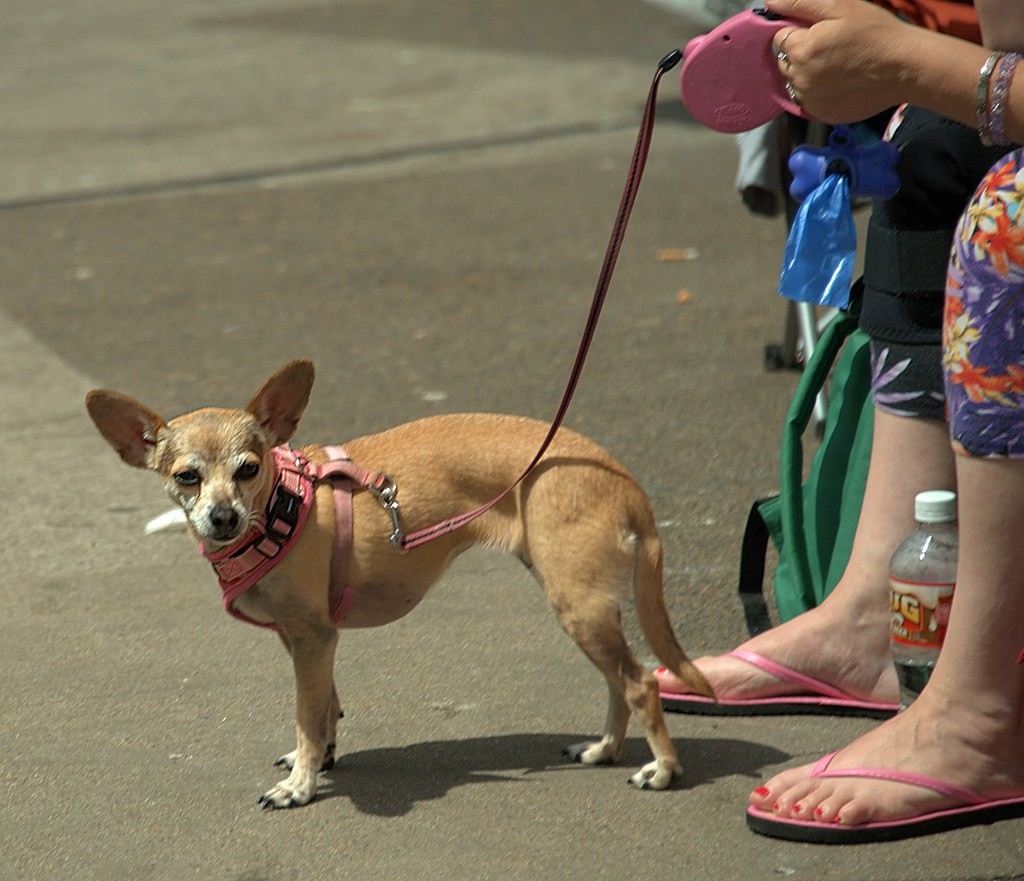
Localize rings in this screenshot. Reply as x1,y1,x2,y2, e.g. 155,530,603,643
785,82,799,105
776,25,803,60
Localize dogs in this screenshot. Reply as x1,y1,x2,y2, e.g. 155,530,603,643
83,357,718,809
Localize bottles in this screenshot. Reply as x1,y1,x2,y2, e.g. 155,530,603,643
888,490,958,711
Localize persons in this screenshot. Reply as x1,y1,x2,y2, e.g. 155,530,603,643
651,0,1024,846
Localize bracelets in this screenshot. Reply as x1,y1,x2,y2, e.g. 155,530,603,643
975,49,1004,147
989,51,1024,144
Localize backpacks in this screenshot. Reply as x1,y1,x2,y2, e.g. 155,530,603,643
739,275,874,639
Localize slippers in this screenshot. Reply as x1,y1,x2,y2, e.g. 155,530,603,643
647,647,903,714
745,749,1024,844
